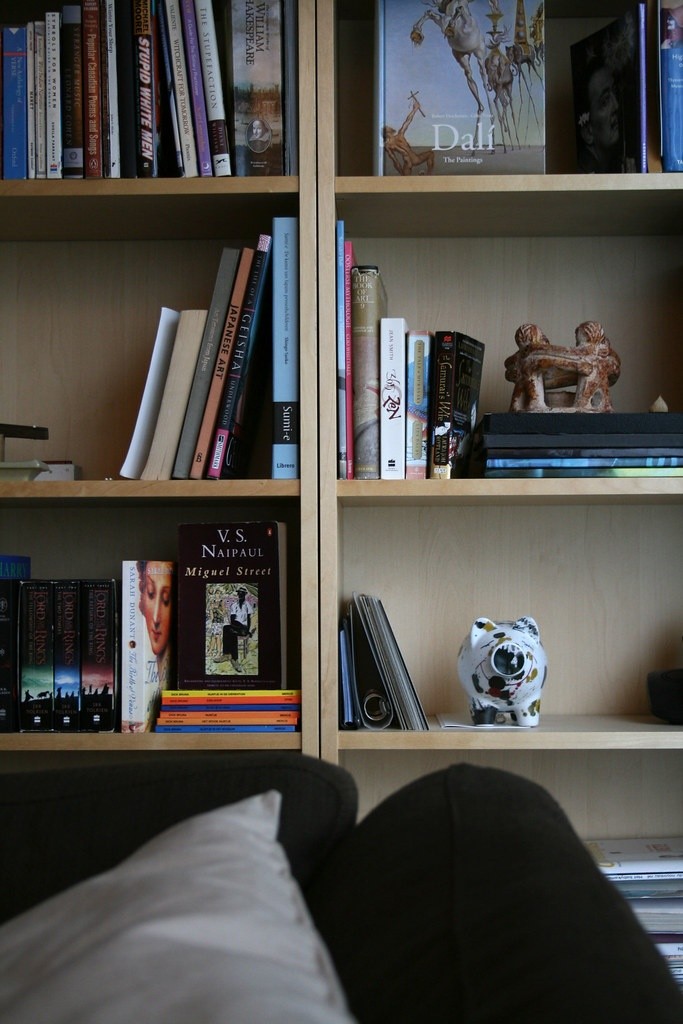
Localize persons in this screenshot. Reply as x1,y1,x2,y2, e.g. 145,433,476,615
136,561,171,691
213,585,254,673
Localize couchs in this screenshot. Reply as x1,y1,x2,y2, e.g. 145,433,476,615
0,751,683,1024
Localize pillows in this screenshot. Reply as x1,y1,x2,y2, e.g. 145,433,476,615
0,790,354,1024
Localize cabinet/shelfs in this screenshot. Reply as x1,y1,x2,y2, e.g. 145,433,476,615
317,0,683,767
0,0,320,765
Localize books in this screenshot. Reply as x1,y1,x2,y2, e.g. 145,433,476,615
0,0,682,733
583,839,683,989
